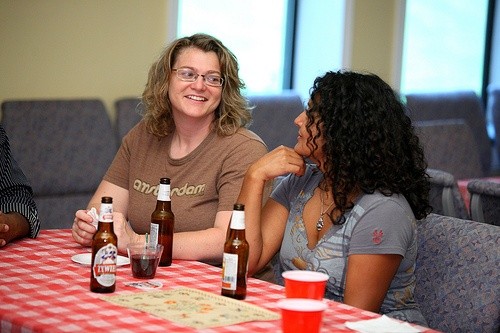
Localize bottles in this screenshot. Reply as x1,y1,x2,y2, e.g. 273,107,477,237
221,204,249,300
90,197,118,294
149,178,175,267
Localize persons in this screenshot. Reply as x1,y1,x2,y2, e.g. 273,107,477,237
226,71,430,326
72,33,273,269
0,122,40,247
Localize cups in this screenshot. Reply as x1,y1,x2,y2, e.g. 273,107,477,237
278,298,328,333
127,244,164,278
281,270,330,300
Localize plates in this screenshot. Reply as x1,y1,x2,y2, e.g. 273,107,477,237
71,253,130,267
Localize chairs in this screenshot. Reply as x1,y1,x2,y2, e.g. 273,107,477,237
4,91,500,332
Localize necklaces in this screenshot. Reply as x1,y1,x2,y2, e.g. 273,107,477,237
316,194,334,231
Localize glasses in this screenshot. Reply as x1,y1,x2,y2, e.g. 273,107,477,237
171,68,225,87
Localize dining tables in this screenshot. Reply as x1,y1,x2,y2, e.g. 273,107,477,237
0,227,442,333
